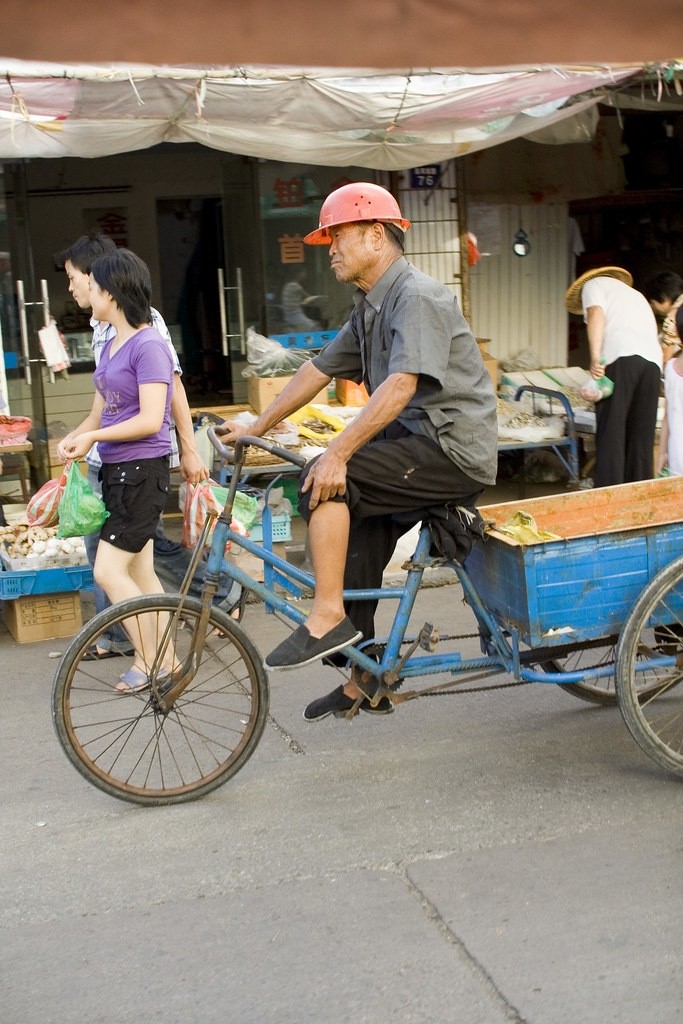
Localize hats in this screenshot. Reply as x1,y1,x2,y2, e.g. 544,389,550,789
565,267,633,315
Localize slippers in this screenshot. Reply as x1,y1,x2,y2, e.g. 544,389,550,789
112,669,177,693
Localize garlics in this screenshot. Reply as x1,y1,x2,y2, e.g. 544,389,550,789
24,536,88,569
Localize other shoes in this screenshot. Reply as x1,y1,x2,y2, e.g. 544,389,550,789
263,615,363,672
302,684,394,723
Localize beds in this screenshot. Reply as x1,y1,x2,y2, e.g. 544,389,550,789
196,385,580,499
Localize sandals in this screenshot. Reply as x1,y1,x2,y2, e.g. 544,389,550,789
81,644,134,661
218,587,249,638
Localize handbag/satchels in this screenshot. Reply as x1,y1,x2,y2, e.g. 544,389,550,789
180,480,251,552
58,461,111,538
575,376,603,402
199,481,257,531
27,459,73,528
595,355,614,398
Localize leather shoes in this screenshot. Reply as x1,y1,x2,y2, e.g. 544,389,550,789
154,537,183,556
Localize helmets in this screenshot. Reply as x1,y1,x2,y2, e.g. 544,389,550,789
303,182,411,244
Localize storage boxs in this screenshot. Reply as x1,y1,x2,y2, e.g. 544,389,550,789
224,513,294,581
501,366,592,398
0,550,97,599
0,591,83,645
31,436,89,481
335,377,370,407
476,337,497,391
248,373,329,416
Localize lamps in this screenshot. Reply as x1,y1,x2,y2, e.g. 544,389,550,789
512,204,530,255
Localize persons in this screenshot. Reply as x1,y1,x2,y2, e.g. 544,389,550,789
565,265,662,489
655,303,683,479
216,181,499,724
276,281,328,336
646,269,683,364
55,233,210,694
